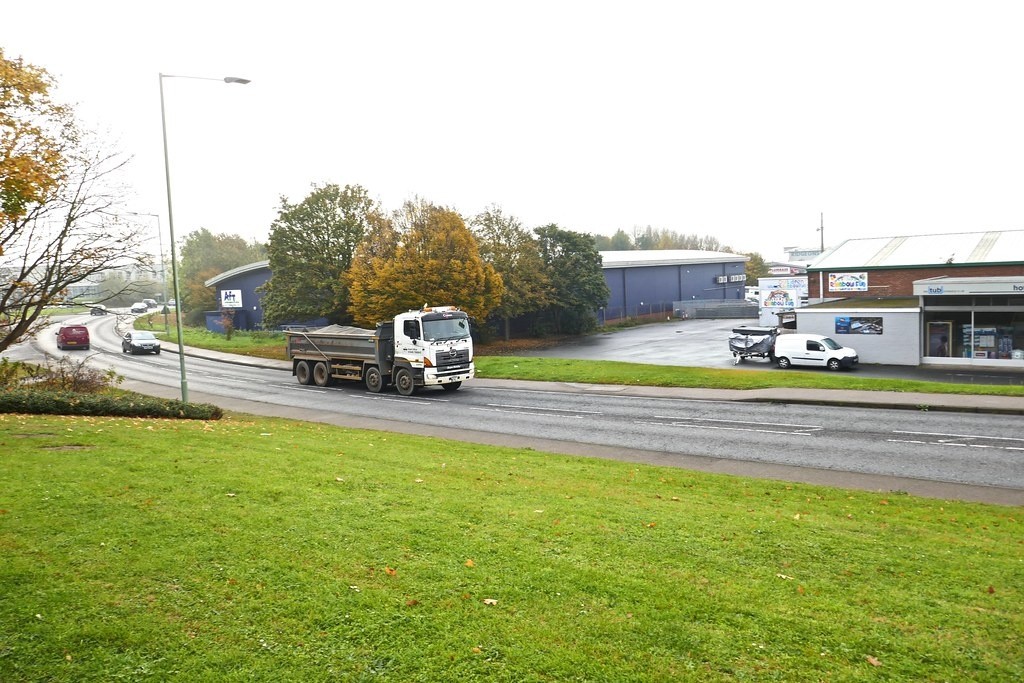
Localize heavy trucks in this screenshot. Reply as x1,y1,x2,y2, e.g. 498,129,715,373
283,305,475,396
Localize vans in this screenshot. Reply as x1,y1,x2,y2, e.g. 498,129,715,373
56,326,90,350
773,335,860,371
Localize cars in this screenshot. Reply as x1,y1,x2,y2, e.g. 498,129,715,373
121,330,160,354
90,304,107,315
142,299,158,308
130,303,147,312
168,299,175,306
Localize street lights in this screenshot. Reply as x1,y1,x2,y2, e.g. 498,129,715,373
157,72,252,404
